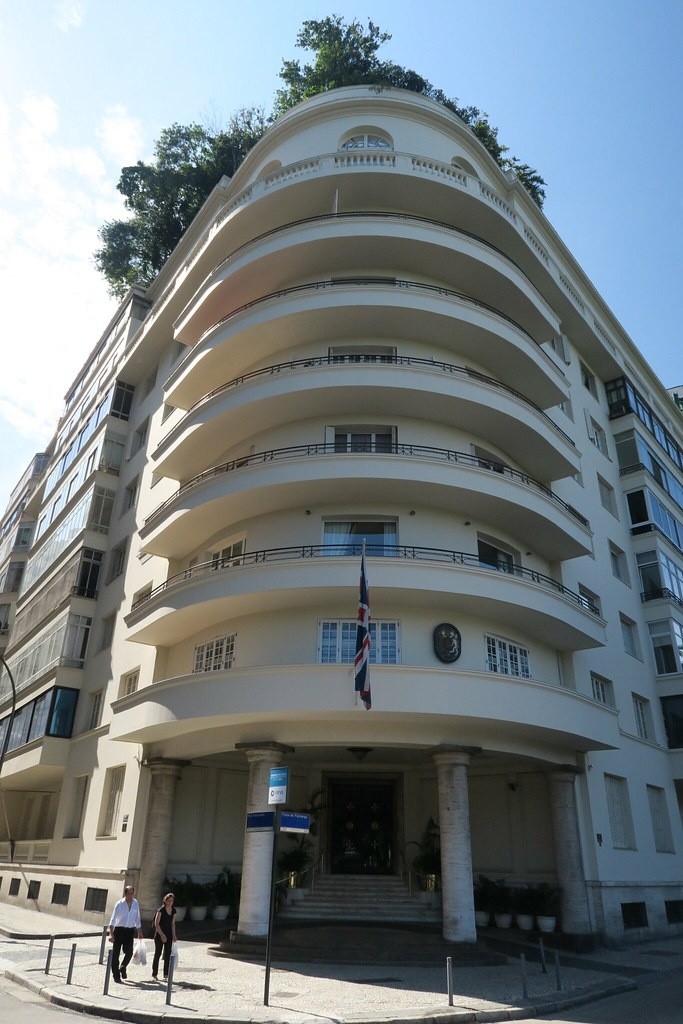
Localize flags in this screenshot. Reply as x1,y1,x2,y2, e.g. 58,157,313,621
354,554,371,711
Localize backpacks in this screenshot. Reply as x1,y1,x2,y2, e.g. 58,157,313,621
152,908,163,928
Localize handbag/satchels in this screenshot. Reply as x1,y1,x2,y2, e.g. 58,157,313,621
132,938,147,966
170,939,178,970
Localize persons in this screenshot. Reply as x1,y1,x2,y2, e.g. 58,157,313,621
109,885,141,983
151,892,177,982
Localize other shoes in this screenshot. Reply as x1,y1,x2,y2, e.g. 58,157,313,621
153,974,159,981
114,976,121,983
164,975,168,978
121,968,127,979
